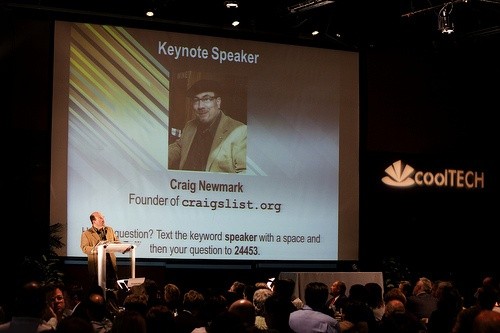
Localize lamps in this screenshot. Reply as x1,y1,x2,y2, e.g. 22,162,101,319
438,10,455,35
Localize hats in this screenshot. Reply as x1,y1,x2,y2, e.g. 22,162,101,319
187,78,222,96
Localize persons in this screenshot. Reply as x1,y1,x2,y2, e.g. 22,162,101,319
168,79,248,173
0,278,500,333
80,211,123,283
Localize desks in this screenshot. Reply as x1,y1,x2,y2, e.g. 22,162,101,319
279,272,385,305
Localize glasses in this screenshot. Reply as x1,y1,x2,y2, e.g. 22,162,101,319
95,215,106,220
191,95,216,105
